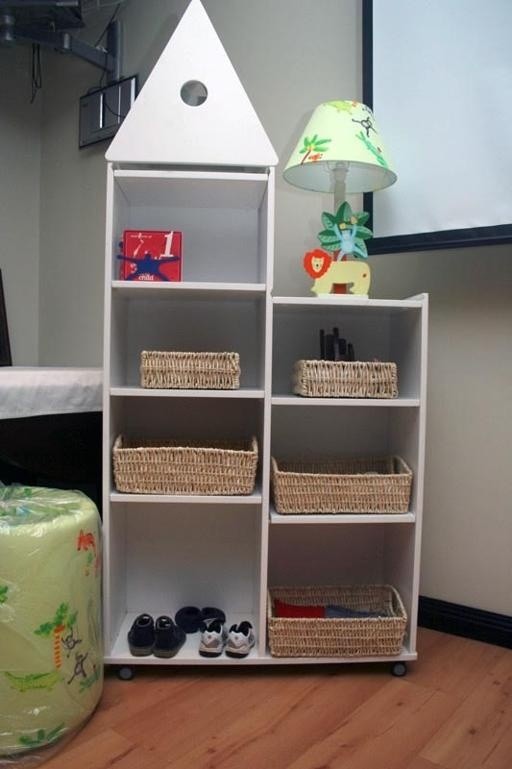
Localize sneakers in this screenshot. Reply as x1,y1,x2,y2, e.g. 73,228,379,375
226,620,255,657
200,606,226,634
128,613,156,657
152,614,187,658
175,606,203,633
199,622,228,658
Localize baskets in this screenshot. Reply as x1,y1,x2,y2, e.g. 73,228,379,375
267,582,408,658
271,453,413,514
110,430,258,495
292,356,401,399
138,349,242,389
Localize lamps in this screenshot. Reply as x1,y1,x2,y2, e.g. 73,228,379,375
278,97,401,296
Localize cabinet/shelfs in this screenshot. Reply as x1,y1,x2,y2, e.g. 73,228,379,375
70,162,431,665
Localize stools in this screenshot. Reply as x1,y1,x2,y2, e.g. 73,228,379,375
0,481,107,759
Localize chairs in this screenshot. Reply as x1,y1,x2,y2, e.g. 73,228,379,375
0,268,105,520
